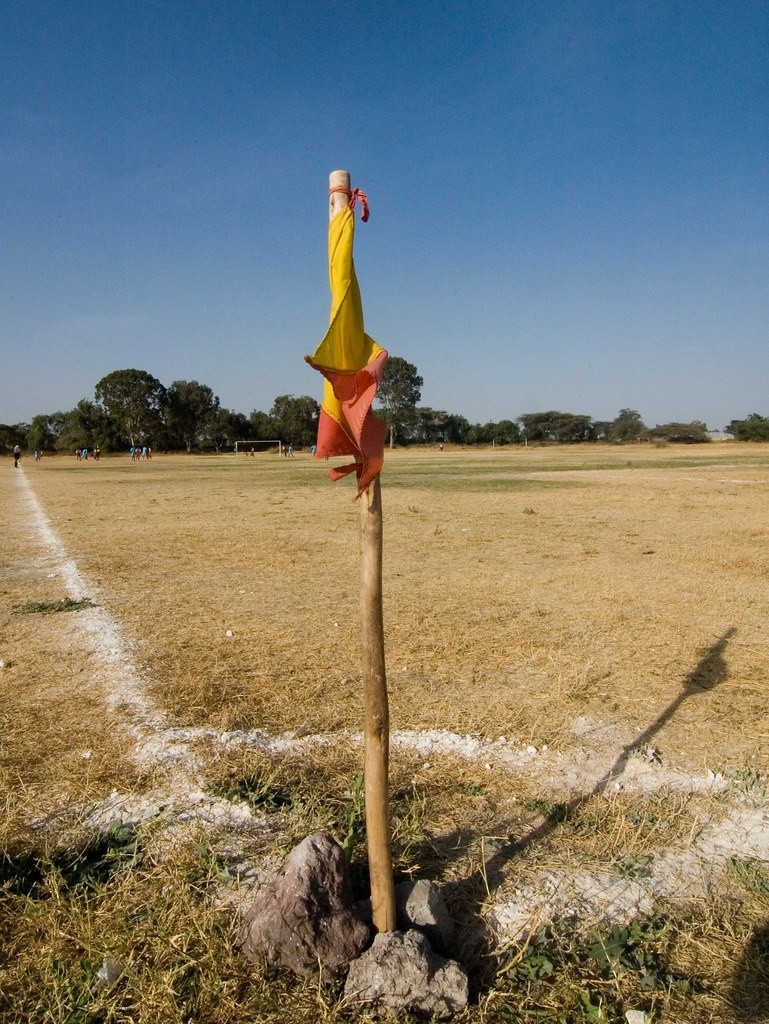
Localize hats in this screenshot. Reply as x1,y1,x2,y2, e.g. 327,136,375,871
16,443,19,445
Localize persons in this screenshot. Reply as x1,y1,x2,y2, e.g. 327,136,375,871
75,445,101,461
249,446,256,457
310,445,316,461
13,443,43,467
283,445,294,458
440,443,443,451
130,446,151,462
